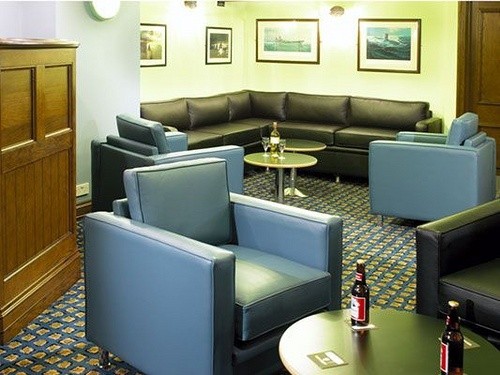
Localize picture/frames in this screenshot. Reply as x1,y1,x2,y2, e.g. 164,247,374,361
256,19,319,64
206,27,232,64
140,23,167,67
358,18,421,74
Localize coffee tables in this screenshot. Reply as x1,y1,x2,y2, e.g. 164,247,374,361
278,308,500,375
268,138,327,197
244,152,318,204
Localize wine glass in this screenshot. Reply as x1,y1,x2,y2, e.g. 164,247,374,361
262,137,269,156
279,139,286,159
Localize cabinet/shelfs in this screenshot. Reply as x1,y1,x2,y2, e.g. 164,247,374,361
0,36,80,345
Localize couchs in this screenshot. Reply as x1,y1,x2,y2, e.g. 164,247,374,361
91,114,244,214
85,158,344,375
369,112,497,223
416,198,500,351
140,90,444,188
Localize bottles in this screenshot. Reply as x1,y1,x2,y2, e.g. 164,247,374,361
270,122,280,158
439,301,464,375
350,259,370,326
